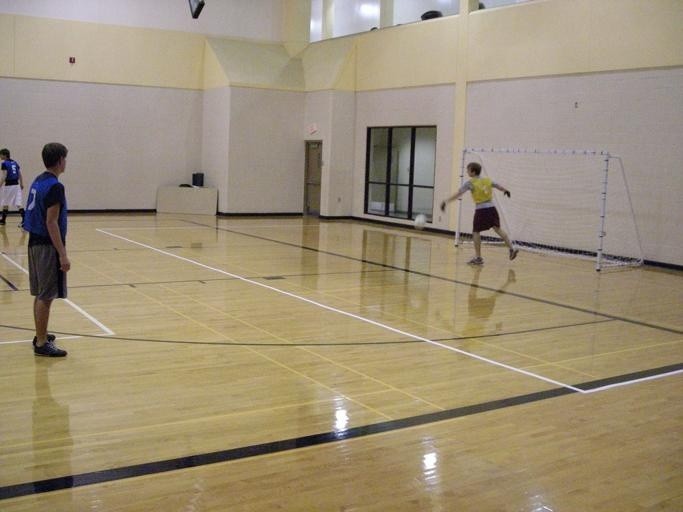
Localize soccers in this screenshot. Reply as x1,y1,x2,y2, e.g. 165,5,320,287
414,214,428,228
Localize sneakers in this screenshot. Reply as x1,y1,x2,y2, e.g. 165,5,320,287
32,335,55,346
0,220,5,225
34,342,67,357
18,221,24,228
509,245,518,260
467,256,484,265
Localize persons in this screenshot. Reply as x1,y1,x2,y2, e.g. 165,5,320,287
0,149,27,227
441,163,519,265
23,142,69,358
